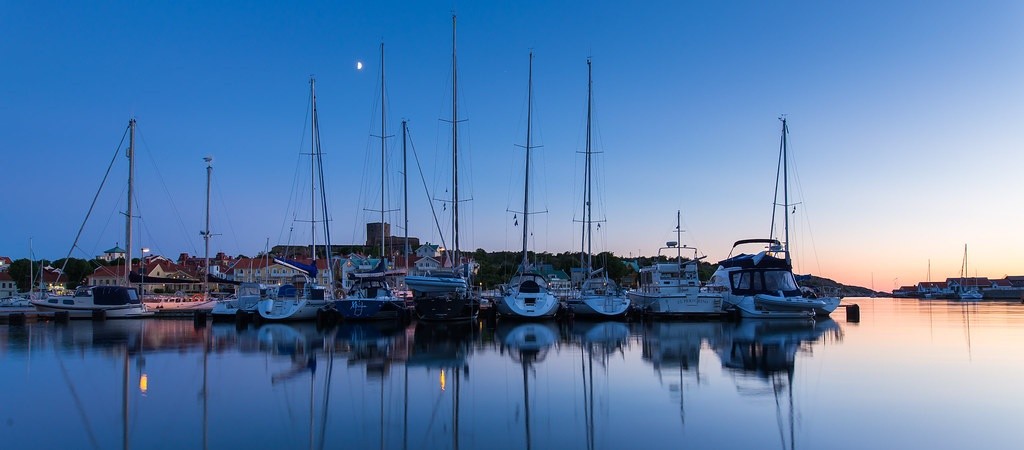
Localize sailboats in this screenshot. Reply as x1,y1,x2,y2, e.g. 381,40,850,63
203,324,804,450
920,244,983,301
0,17,840,324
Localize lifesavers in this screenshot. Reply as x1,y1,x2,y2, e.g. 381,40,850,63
334,289,346,300
